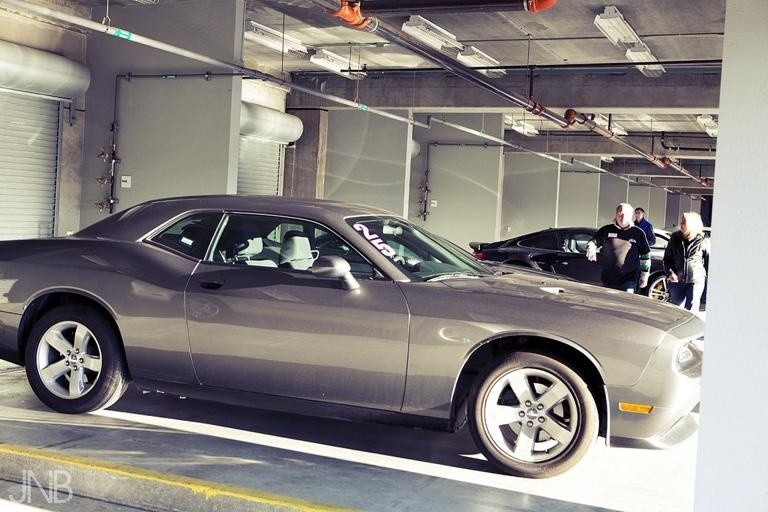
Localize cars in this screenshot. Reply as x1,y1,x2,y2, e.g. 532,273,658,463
1,190,707,480
469,222,709,311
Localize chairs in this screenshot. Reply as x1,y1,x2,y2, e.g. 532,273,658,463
561,233,580,254
229,220,278,268
277,231,320,270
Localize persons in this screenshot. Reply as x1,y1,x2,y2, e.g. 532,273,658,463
633,207,657,248
664,212,710,313
586,202,652,294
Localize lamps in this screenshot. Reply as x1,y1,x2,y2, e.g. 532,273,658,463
241,4,721,139
